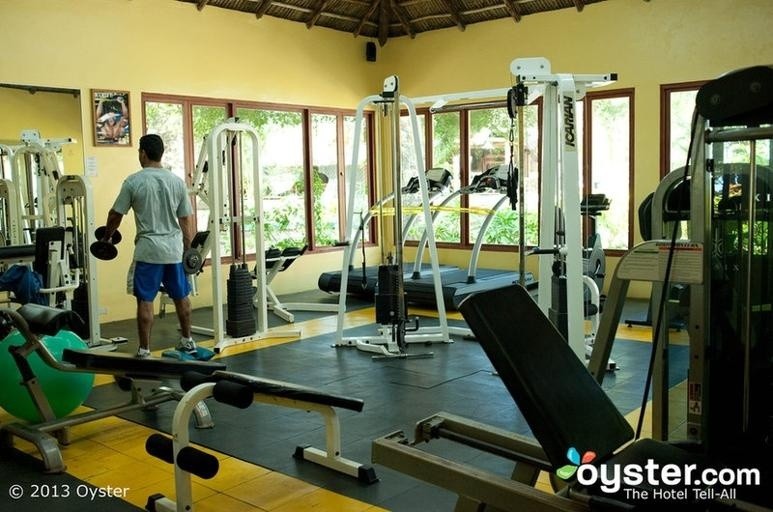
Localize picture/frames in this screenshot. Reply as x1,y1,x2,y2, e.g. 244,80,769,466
89,88,132,147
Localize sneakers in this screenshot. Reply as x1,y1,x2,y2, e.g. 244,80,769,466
175,339,197,354
134,351,151,359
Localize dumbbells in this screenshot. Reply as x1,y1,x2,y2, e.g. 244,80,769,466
89,226,122,260
181,248,203,274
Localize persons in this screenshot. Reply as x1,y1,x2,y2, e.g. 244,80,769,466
98,133,198,359
94,96,128,141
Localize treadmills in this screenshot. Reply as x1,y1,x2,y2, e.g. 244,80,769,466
317,168,461,299
402,165,535,311
